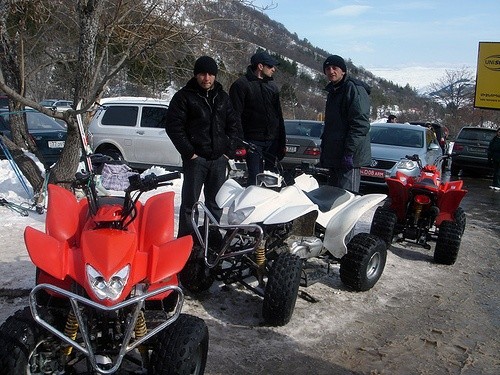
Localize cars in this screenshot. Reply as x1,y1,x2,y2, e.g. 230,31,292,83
403,121,450,172
0,110,83,163
359,123,449,196
280,120,329,183
450,124,498,180
39,99,74,110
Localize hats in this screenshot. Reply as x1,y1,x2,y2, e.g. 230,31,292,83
323,55,346,75
194,55,218,75
250,52,281,67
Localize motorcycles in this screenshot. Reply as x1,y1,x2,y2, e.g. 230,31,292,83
0,169,210,375
179,135,397,327
370,152,469,266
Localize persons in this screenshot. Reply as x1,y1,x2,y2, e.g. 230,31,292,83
321,55,372,192
165,56,239,237
488,128,500,190
229,52,286,188
387,115,397,123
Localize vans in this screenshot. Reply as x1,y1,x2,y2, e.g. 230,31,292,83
85,97,184,172
